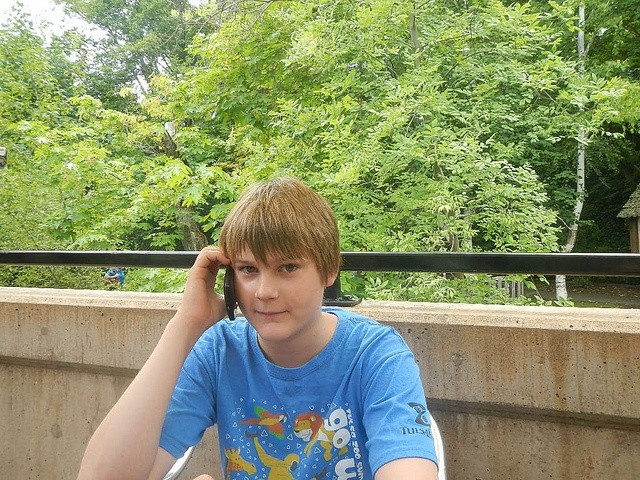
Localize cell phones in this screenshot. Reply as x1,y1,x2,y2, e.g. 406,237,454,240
223,265,237,324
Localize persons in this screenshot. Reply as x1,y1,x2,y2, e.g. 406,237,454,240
74,177,439,480
